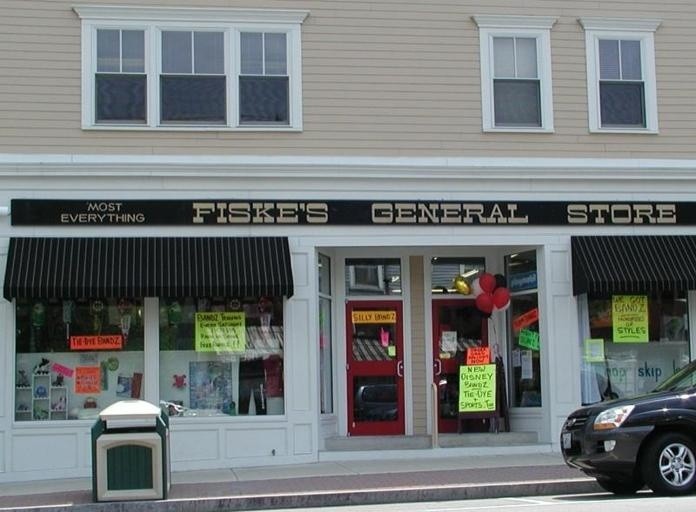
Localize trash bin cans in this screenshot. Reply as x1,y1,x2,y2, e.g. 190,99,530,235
91,400,170,502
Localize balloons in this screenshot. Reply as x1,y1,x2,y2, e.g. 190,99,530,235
493,273,507,288
493,286,511,311
493,299,511,313
479,272,496,293
476,293,493,316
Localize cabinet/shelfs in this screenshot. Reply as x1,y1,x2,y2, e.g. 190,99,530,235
15,373,69,420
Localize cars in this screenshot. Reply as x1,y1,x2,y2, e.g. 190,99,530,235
560,360,696,497
